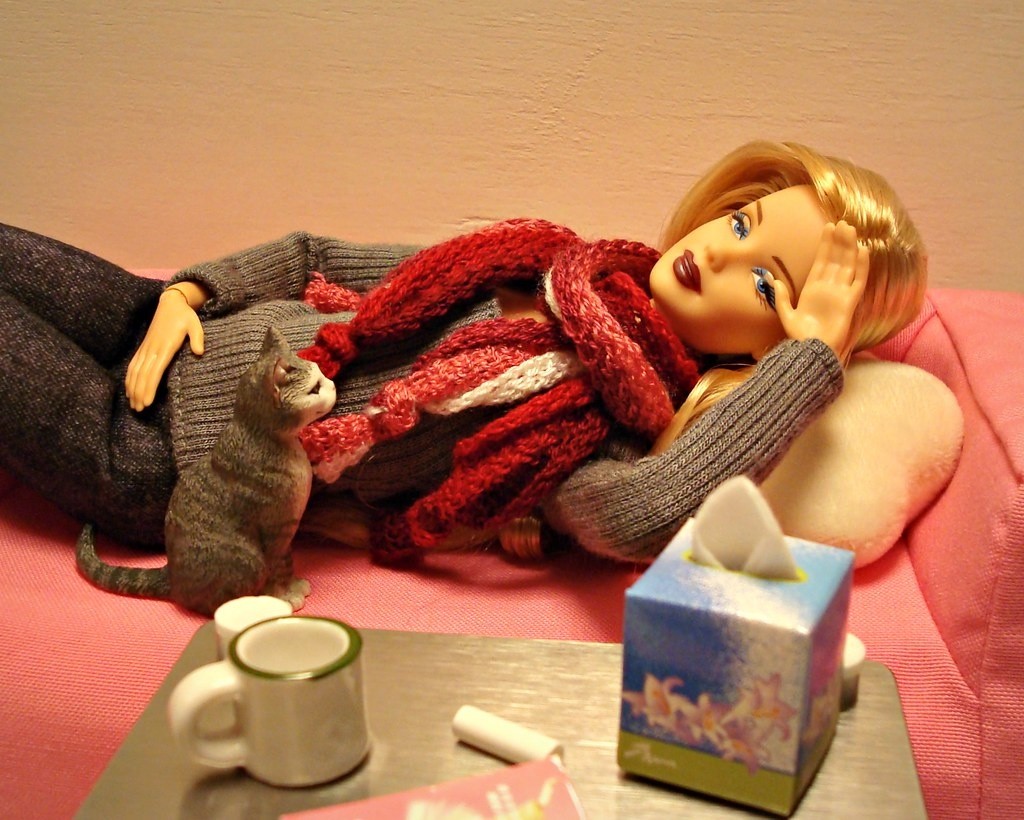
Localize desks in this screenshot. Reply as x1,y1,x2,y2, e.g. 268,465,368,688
72,617,930,820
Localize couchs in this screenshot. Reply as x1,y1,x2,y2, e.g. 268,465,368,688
1,287,1023,820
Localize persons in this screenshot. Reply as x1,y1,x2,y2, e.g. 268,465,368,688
0,140,923,558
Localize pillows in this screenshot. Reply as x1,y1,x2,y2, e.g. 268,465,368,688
758,362,965,568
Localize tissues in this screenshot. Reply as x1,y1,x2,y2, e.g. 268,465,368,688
615,470,857,818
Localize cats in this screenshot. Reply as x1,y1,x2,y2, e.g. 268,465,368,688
72,328,339,615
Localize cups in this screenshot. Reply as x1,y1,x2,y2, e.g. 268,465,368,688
168,616,370,787
